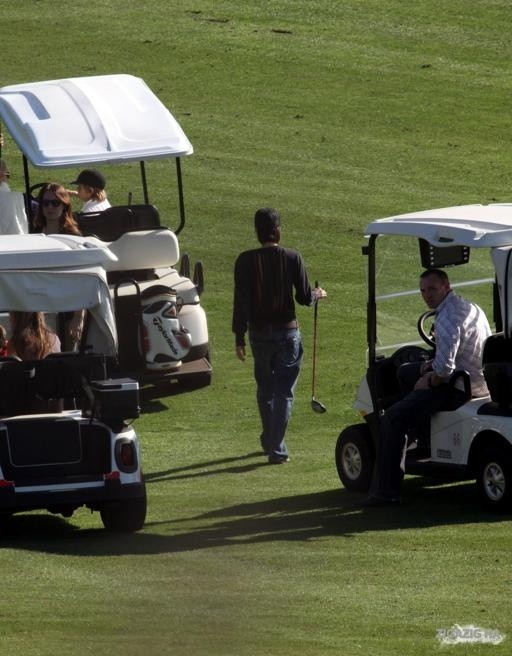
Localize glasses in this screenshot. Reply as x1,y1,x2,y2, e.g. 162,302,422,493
2,172,9,177
38,197,63,209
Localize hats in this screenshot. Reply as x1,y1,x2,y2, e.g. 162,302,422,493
253,207,281,229
68,168,106,190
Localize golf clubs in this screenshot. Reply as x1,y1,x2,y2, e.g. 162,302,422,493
312,279,327,414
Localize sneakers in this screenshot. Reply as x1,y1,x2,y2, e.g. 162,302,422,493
354,494,400,507
262,444,287,462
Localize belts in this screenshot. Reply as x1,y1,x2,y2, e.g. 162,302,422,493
268,318,298,330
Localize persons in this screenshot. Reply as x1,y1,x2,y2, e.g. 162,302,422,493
29,180,79,235
67,169,113,215
229,204,329,466
8,311,61,360
0,325,18,358
347,268,494,513
